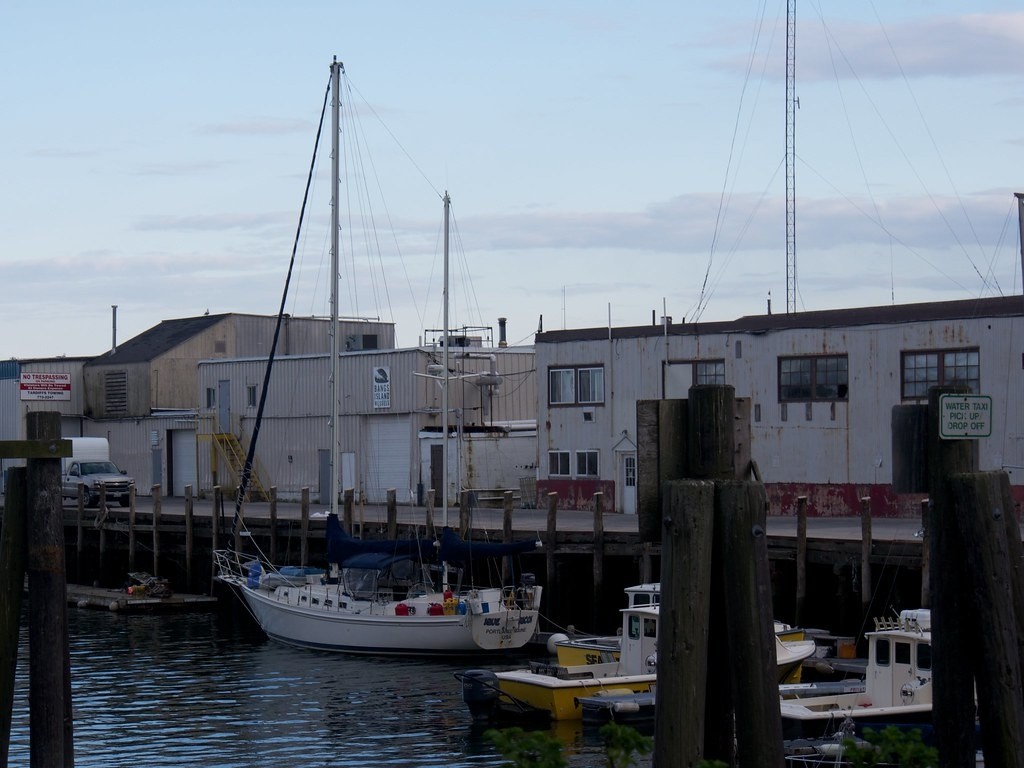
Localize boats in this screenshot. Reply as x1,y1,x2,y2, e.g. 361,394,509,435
213,56,543,652
573,683,656,725
23,571,218,612
780,610,934,732
462,606,815,723
554,581,804,682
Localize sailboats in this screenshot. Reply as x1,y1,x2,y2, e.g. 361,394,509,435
302,189,603,656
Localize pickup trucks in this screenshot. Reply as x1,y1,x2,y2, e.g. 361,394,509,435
61,437,134,508
